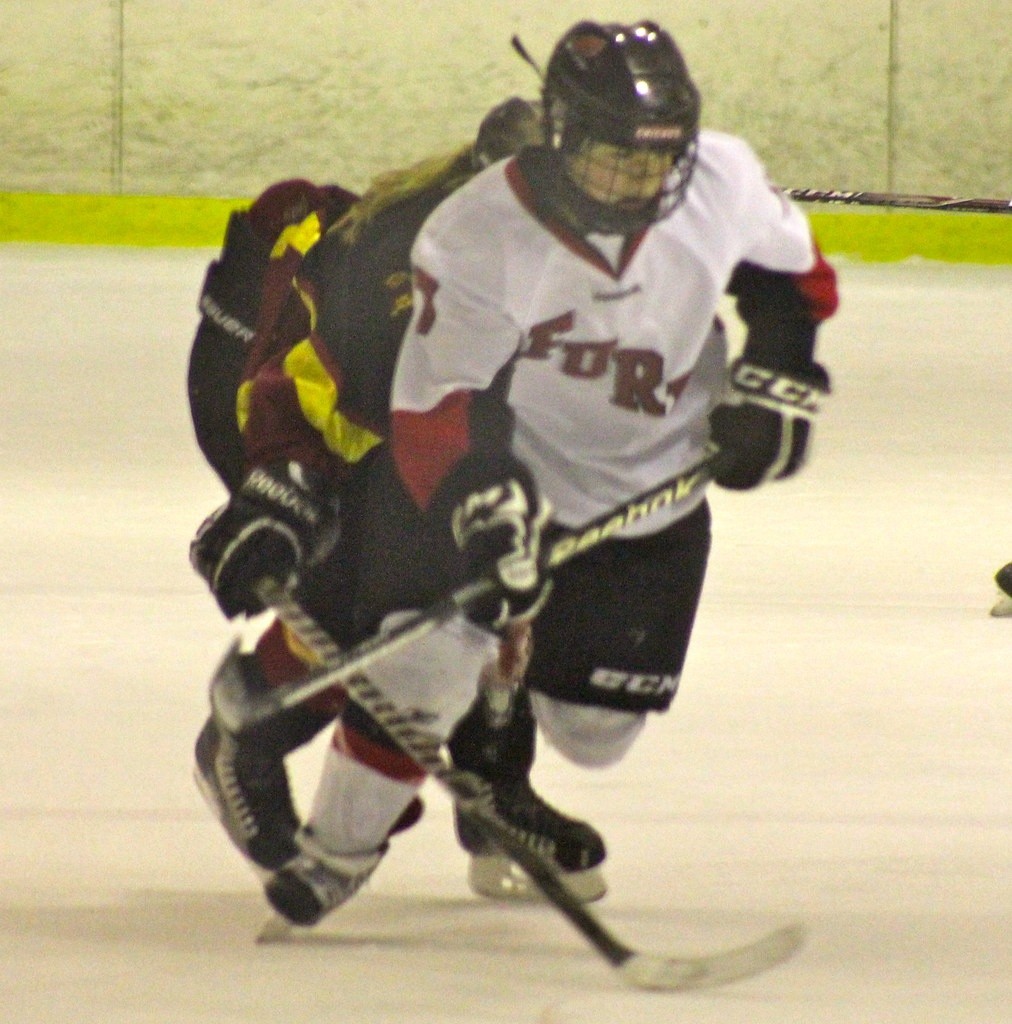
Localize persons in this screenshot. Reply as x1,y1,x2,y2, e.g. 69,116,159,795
188,17,840,926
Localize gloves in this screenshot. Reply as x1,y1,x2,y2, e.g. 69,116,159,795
706,356,829,493
426,446,554,633
189,465,341,621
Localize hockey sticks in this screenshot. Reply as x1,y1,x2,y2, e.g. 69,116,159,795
248,573,813,998
209,452,730,737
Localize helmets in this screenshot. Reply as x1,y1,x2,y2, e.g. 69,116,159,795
542,19,702,233
477,98,544,161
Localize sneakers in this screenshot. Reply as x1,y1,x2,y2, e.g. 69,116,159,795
253,796,423,943
450,719,607,906
191,654,337,883
